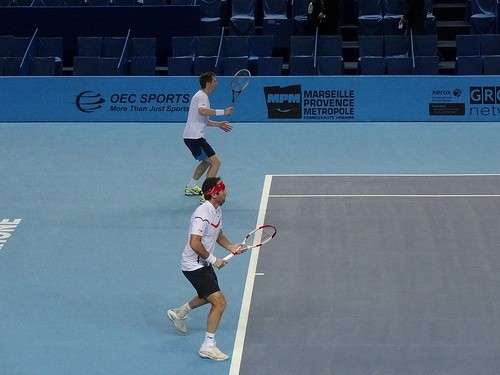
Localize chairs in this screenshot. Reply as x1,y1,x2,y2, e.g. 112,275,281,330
0,0,500,76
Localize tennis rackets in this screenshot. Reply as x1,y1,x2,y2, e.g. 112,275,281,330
228,69,250,117
223,225,276,260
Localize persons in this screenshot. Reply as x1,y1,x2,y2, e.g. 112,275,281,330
183,72,234,206
167,177,247,361
304,0,327,36
402,0,428,36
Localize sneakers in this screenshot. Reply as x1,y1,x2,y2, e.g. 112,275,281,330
199,344,230,362
184,185,206,205
167,308,188,333
